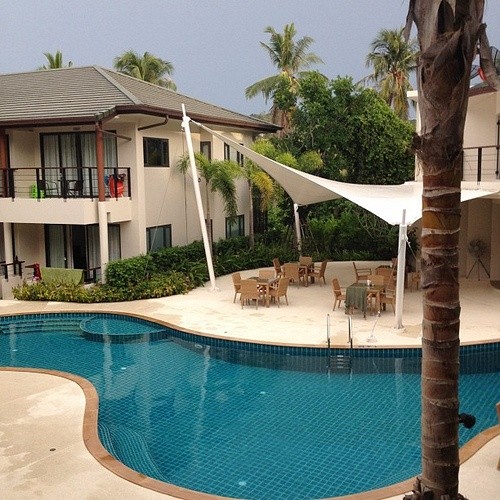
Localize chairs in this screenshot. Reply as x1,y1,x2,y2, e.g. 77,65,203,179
232,256,421,319
38,180,59,198
65,179,84,198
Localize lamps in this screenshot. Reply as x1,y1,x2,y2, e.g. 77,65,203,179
458,411,476,429
